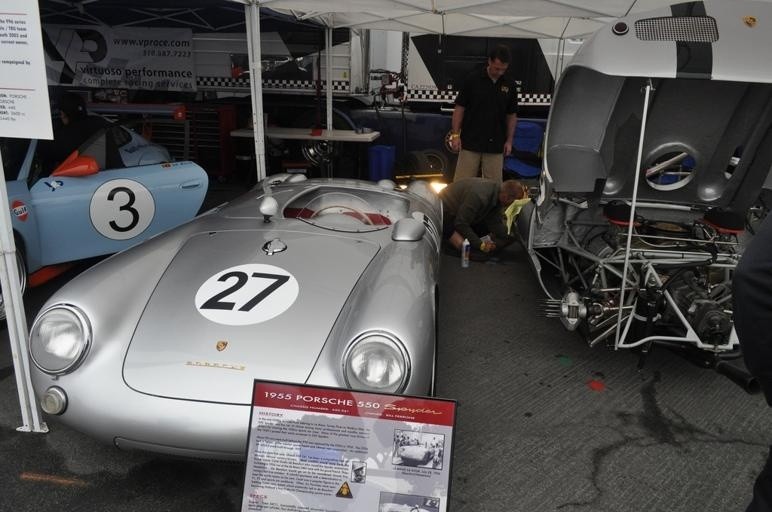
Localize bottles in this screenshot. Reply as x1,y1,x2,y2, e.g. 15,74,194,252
461,238,470,269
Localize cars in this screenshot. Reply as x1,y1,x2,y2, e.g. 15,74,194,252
1,110,208,320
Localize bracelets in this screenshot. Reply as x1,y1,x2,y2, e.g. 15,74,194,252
452,132,461,140
479,242,485,251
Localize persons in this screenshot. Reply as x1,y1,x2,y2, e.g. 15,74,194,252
451,46,518,182
39,92,126,177
437,177,524,261
732,209,772,512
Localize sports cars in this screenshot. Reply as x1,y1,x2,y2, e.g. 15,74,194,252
27,172,446,463
